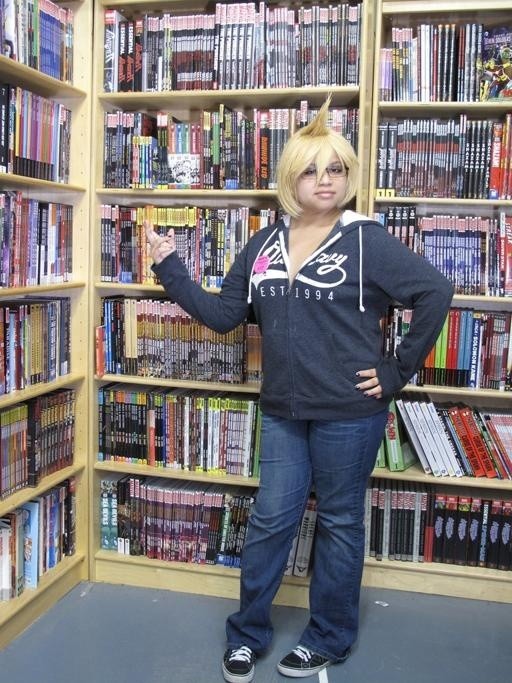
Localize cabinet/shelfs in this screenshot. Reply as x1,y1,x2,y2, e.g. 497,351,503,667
89,0,374,610
363,0,512,610
0,0,94,650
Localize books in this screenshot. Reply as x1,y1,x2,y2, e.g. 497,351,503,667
0,0,78,602
99,2,359,575
365,21,510,573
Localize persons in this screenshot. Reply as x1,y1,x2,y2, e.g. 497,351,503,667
144,94,454,683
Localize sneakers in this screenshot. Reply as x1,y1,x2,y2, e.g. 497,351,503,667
223,643,256,683
278,646,333,678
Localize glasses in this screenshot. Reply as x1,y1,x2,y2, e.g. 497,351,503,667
301,164,344,180
